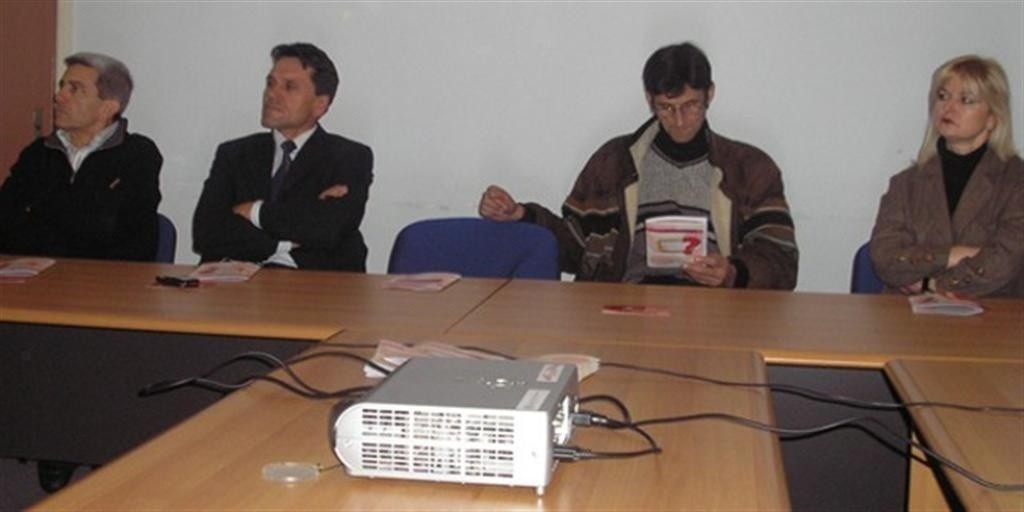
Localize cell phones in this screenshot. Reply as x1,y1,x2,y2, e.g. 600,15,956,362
155,273,200,289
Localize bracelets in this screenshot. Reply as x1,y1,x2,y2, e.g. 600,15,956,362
920,276,930,293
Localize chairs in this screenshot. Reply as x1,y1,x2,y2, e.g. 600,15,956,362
849,241,882,292
152,213,177,262
389,217,560,279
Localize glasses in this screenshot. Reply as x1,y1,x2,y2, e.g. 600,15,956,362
651,100,710,120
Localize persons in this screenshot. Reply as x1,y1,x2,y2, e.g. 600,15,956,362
478,41,801,292
865,54,1024,299
0,49,164,263
188,42,374,275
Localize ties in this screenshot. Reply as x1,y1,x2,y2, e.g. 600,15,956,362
268,141,297,199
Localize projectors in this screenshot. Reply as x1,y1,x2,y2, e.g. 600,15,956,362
327,354,582,495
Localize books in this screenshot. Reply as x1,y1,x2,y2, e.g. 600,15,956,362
0,253,55,278
908,293,985,318
378,269,462,294
642,215,709,270
364,339,600,383
187,257,260,283
